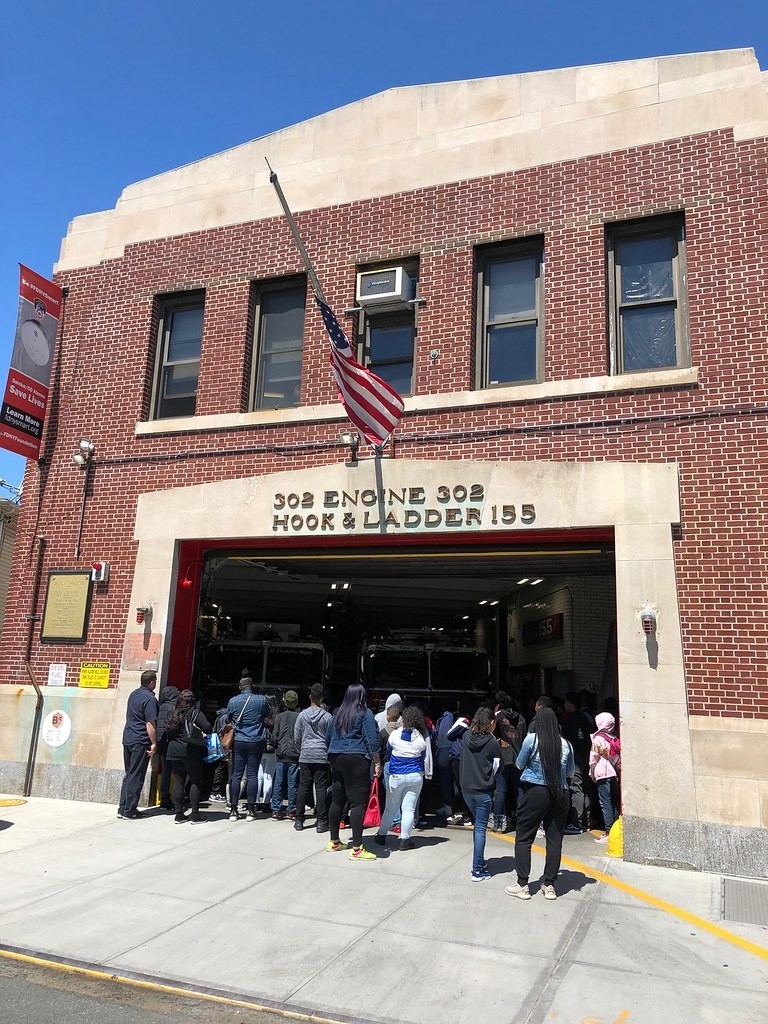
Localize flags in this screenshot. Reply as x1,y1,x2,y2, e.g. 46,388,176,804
314,294,405,452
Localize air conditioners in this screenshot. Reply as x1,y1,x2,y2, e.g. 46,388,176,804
355,267,412,314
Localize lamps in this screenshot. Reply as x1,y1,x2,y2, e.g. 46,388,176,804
341,431,360,461
70,438,95,465
137,604,152,624
642,611,656,635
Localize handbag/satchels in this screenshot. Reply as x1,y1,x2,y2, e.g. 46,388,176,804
204,732,225,763
218,724,235,749
183,709,207,747
362,776,381,826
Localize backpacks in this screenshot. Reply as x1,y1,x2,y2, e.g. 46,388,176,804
594,733,621,776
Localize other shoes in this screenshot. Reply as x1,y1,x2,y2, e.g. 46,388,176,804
294,823,303,831
117,808,148,820
565,821,590,833
271,809,296,820
595,834,608,844
374,834,386,845
436,804,453,816
447,811,472,826
208,794,226,802
317,824,331,833
399,839,415,851
470,870,491,882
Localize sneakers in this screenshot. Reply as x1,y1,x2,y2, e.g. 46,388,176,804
174,811,208,824
504,883,531,900
540,882,557,900
229,809,258,821
325,839,349,851
349,844,377,860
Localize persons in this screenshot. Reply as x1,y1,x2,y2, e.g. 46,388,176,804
504,708,576,900
163,689,212,824
294,683,333,832
207,688,618,837
324,683,383,861
374,706,433,851
268,690,302,821
458,707,508,882
116,670,157,820
156,686,182,809
589,712,617,844
224,677,271,822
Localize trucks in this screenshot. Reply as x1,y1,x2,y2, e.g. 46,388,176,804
197,601,337,729
353,618,499,727
